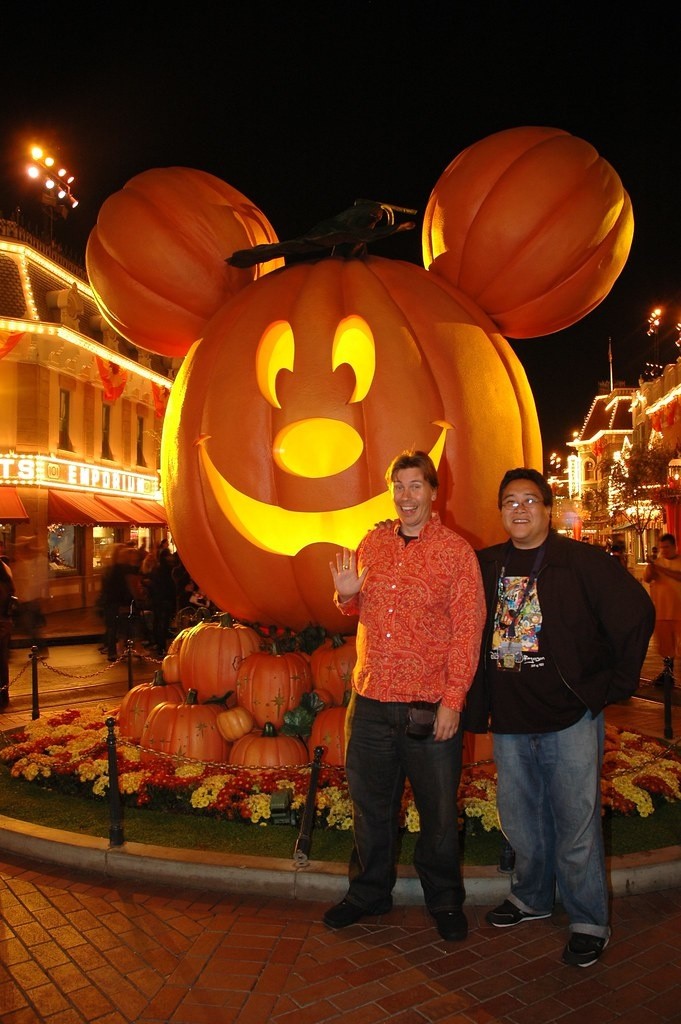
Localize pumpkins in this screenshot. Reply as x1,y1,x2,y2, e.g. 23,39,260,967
422,126,634,340
114,609,497,780
85,166,284,358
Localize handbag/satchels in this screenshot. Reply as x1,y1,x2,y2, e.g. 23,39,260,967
406,708,436,739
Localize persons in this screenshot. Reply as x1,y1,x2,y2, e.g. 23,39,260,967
581,534,626,566
646,544,657,563
365,466,660,969
643,533,681,688
322,447,485,942
0,530,196,706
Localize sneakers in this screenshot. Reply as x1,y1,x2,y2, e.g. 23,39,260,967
325,897,393,928
485,900,553,927
563,926,611,969
431,903,469,941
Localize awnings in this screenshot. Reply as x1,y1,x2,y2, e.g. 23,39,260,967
0,485,30,541
46,488,128,527
612,520,637,531
132,497,171,525
598,523,621,535
92,492,168,533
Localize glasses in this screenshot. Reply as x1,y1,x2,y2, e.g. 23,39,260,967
499,497,542,510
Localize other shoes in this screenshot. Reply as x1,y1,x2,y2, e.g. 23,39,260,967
660,667,673,677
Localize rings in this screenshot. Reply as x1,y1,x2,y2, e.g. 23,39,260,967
343,565,349,569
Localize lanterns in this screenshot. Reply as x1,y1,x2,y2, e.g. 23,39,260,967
86,124,636,634
